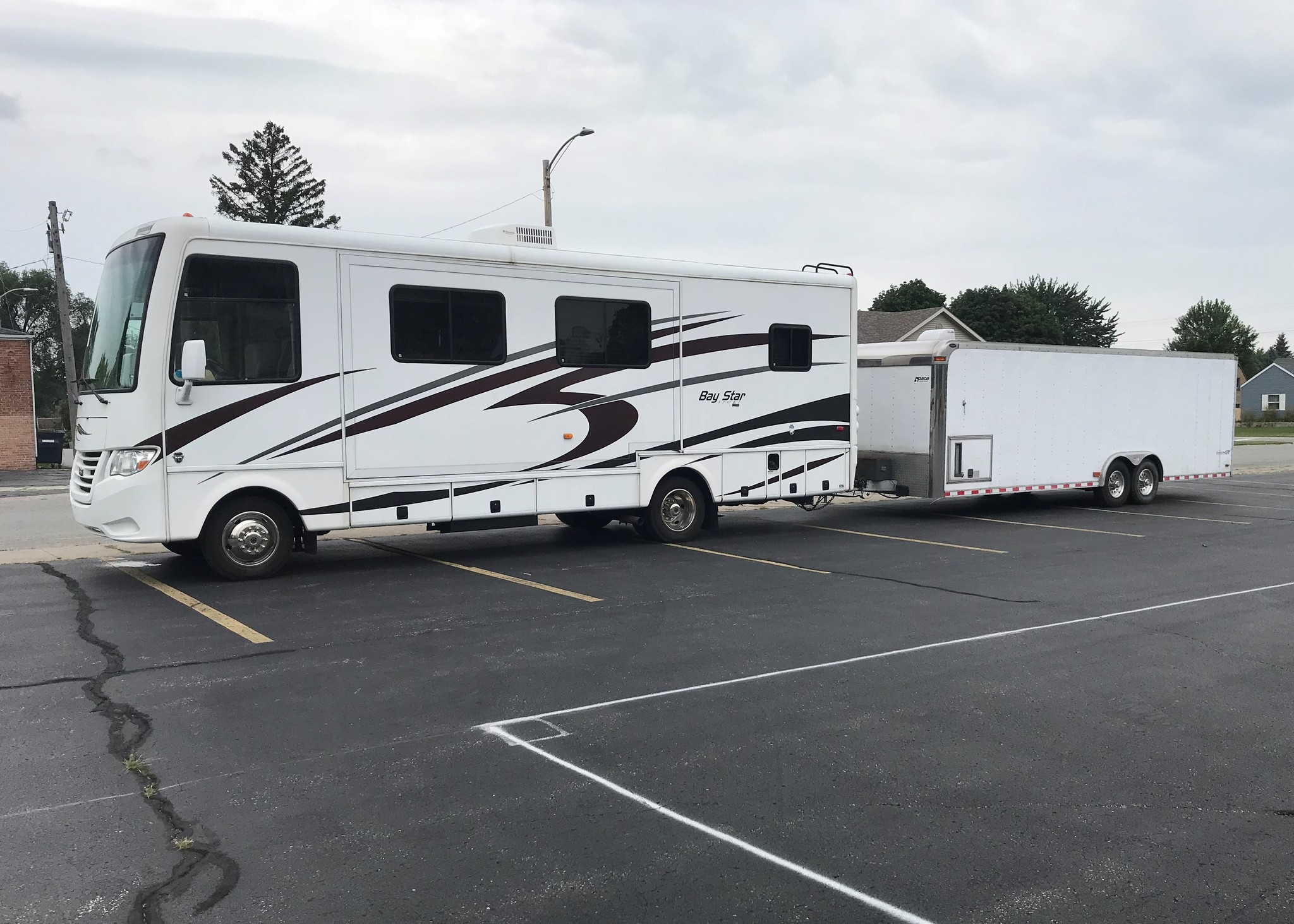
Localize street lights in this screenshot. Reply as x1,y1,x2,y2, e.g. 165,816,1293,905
541,126,597,227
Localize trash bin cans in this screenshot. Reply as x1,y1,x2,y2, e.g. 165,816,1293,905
36,427,64,464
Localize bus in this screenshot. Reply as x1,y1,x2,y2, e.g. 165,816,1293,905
65,208,862,582
